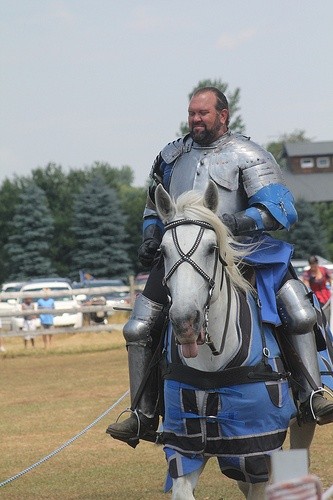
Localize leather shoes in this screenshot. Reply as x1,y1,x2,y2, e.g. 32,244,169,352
106,411,159,438
299,393,333,424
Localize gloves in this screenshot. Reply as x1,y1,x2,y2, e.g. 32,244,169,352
219,213,236,235
138,239,158,266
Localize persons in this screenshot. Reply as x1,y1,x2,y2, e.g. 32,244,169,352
104,87,333,440
302,255,331,309
21,293,39,351
38,288,57,349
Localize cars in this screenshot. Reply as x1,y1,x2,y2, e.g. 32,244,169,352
0,270,150,316
6,281,87,335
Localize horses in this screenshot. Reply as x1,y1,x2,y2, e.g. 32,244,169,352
155,177,320,499
